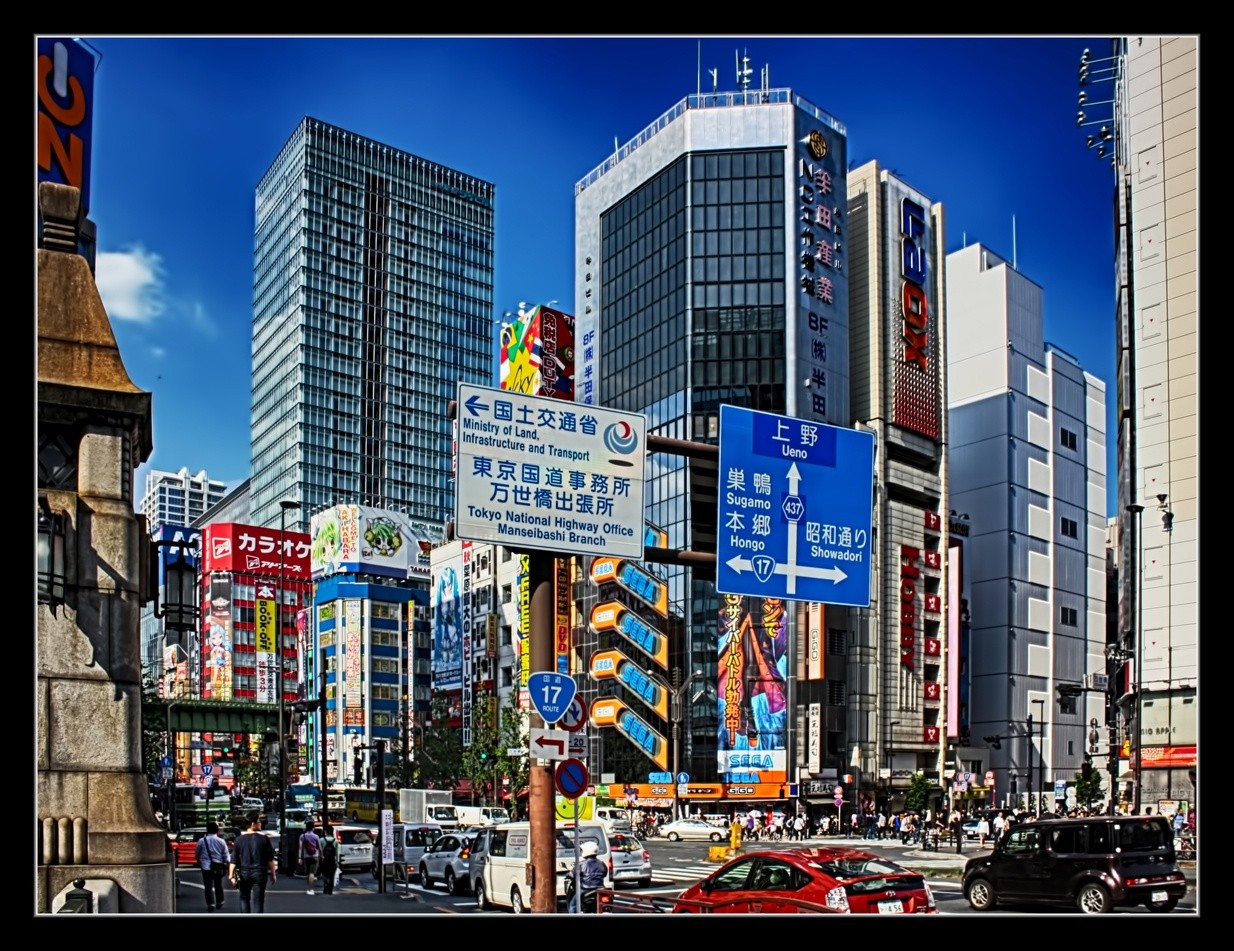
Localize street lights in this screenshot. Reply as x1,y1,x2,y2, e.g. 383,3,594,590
276,496,303,875
886,721,901,821
1125,502,1146,815
168,689,200,834
1031,699,1045,818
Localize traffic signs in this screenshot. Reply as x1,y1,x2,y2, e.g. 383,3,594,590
456,382,645,561
717,405,875,608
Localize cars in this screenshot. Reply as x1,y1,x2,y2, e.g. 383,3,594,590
608,834,652,887
658,818,731,842
671,846,939,914
275,807,308,830
418,832,495,895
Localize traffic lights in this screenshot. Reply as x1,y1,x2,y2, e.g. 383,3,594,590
984,734,1003,749
477,753,497,760
354,757,363,785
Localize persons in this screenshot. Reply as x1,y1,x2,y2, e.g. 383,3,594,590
195,823,231,910
227,813,276,914
634,802,1197,850
299,822,341,895
565,841,608,914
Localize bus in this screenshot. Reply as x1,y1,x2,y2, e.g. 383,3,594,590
150,782,234,825
344,788,404,824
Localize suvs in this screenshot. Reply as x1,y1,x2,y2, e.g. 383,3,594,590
960,814,1188,914
962,809,1018,841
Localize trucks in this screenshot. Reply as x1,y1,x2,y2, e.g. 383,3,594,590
400,786,511,829
555,795,634,838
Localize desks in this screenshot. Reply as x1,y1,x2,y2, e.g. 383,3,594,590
1176,833,1197,850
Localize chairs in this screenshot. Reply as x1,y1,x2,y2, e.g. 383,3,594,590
755,866,793,892
1023,835,1086,851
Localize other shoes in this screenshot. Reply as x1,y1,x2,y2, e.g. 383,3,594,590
307,889,315,896
208,905,215,912
323,888,333,895
217,901,224,909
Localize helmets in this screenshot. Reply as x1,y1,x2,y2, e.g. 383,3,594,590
579,841,599,857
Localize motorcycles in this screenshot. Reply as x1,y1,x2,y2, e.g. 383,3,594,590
560,861,614,915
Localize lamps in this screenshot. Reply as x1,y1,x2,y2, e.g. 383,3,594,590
1157,490,1173,530
1076,48,1120,160
149,528,204,647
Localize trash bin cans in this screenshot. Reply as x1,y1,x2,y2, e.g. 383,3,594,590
285,827,305,873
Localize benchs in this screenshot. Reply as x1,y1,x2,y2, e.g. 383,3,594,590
170,842,202,865
1172,845,1197,858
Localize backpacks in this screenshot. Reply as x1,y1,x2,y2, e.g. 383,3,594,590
323,836,336,859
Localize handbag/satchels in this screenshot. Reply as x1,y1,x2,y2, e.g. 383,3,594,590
304,839,316,855
211,862,227,880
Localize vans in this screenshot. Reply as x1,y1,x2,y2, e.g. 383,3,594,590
235,796,266,814
469,821,616,914
314,826,444,883
